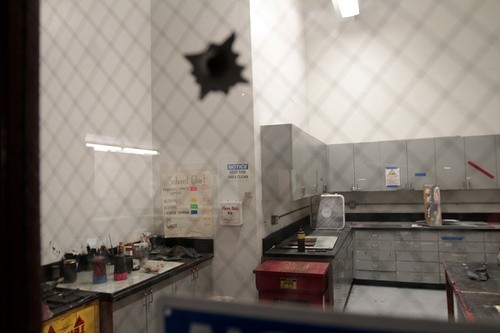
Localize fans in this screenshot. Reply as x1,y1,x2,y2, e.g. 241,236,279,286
310,194,345,231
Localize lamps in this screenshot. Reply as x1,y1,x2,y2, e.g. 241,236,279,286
86,135,157,156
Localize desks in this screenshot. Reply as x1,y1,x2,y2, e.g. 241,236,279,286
442,261,500,326
253,260,330,309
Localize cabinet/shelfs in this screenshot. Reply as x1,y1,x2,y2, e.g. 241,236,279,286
99,276,173,333
329,142,381,192
435,135,496,190
173,259,213,301
352,229,500,290
260,124,329,202
379,138,437,191
264,231,354,313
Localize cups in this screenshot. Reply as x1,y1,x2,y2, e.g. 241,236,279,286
63,259,78,283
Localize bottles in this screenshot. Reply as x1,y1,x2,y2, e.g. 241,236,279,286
297,225,305,252
51,266,60,280
